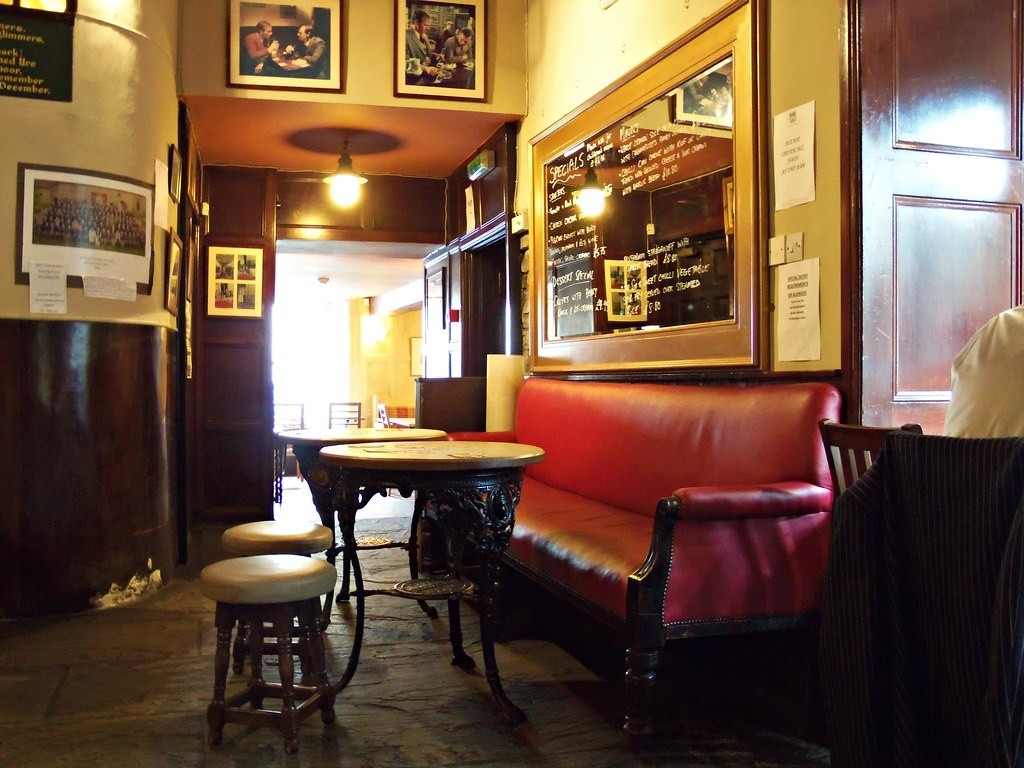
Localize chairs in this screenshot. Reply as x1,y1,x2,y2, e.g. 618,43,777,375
817,416,925,504
329,401,362,429
273,403,305,483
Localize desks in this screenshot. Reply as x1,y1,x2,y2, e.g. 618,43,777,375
279,426,445,619
316,441,547,728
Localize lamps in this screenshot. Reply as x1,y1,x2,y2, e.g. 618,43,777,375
570,166,612,219
322,139,368,211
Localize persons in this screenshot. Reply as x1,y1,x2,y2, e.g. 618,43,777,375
685,73,734,116
243,19,279,76
405,9,473,77
36,195,145,249
286,23,327,77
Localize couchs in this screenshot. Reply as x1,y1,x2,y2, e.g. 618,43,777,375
420,375,844,756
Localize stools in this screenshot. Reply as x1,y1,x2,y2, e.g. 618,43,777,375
198,519,341,756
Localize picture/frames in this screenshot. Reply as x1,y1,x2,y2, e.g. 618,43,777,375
409,336,423,377
225,0,346,96
392,0,489,104
524,0,769,373
426,266,447,331
722,174,735,235
163,224,184,317
167,144,183,205
671,54,739,132
204,243,265,321
